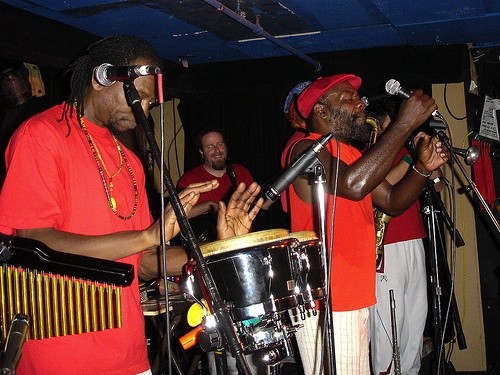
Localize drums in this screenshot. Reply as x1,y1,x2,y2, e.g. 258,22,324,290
289,229,328,304
196,227,314,323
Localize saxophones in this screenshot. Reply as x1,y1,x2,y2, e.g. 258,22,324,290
362,116,392,267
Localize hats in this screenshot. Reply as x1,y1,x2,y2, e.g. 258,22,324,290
289,74,362,130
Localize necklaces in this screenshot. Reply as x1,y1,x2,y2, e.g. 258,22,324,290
73,94,139,221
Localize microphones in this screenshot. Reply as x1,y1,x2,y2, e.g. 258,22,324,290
0,313,31,375
384,79,443,118
94,62,160,86
265,132,334,203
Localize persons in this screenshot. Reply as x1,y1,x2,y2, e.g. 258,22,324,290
288,71,449,375
0,35,264,375
173,128,258,244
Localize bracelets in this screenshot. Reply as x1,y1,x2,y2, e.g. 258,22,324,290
411,163,432,177
